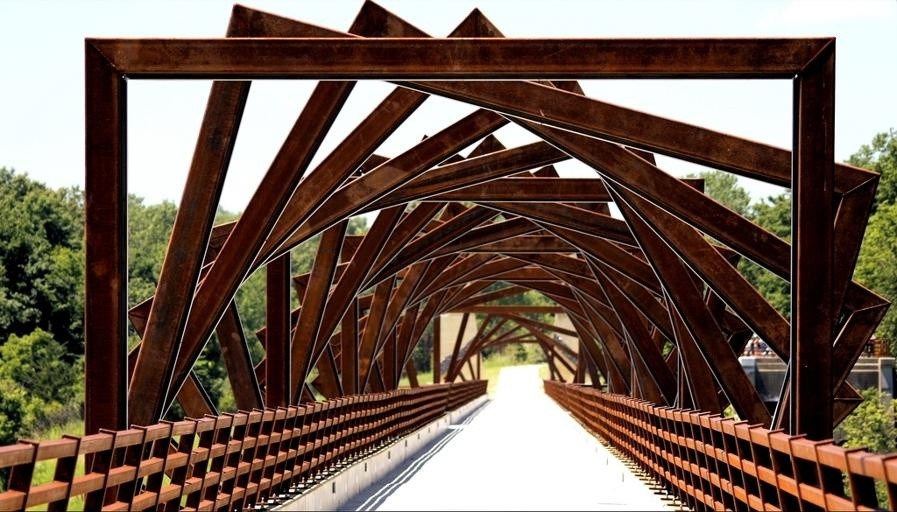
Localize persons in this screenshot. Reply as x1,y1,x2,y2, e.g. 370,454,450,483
750,336,760,355
865,340,875,357
